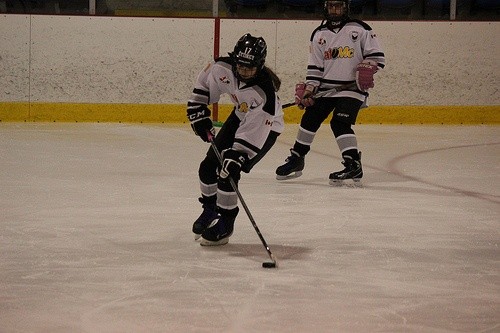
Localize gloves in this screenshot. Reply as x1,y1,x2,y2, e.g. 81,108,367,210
355,60,378,92
191,117,216,143
294,83,318,110
216,147,249,183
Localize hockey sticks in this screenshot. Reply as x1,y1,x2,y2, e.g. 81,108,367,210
211,83,356,127
207,132,277,267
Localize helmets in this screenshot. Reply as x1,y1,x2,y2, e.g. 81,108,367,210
323,0,350,21
232,33,267,83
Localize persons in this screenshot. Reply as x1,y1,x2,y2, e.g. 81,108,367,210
275,0,386,180
186,33,284,242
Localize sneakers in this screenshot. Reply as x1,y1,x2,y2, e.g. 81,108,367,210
329,151,367,188
275,148,304,180
192,194,240,247
